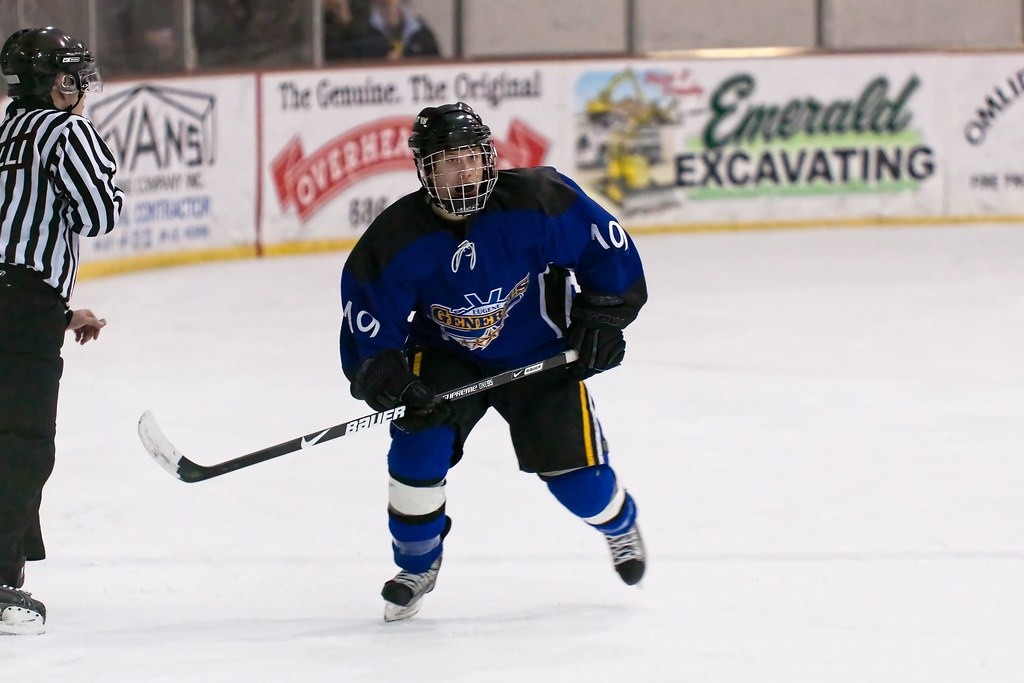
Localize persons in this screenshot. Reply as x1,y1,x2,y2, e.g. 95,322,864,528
339,103,647,624
0,24,127,635
106,0,442,84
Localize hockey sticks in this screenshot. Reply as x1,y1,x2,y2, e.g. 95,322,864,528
138,350,581,485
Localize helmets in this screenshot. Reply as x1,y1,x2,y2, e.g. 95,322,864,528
407,101,492,176
0,26,96,100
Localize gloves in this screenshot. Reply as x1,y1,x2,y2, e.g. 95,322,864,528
347,348,456,433
559,289,637,384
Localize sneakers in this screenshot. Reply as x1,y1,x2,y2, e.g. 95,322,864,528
1,585,47,636
606,523,646,585
381,554,444,621
